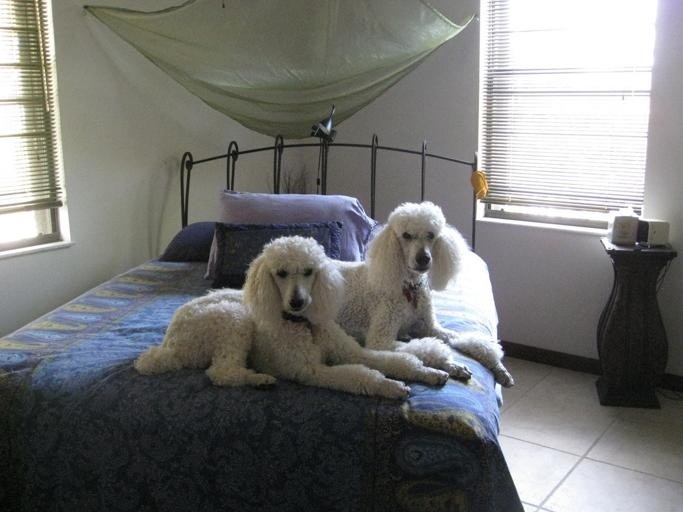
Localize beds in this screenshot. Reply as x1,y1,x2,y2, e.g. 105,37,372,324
0,106,522,507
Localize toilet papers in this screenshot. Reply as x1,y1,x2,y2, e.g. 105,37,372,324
609,207,639,246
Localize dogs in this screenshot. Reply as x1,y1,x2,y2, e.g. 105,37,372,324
322,201,517,389
132,233,451,402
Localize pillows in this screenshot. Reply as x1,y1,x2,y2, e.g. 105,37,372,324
205,189,377,280
213,220,343,288
159,220,216,264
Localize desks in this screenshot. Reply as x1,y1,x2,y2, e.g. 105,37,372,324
597,238,676,408
